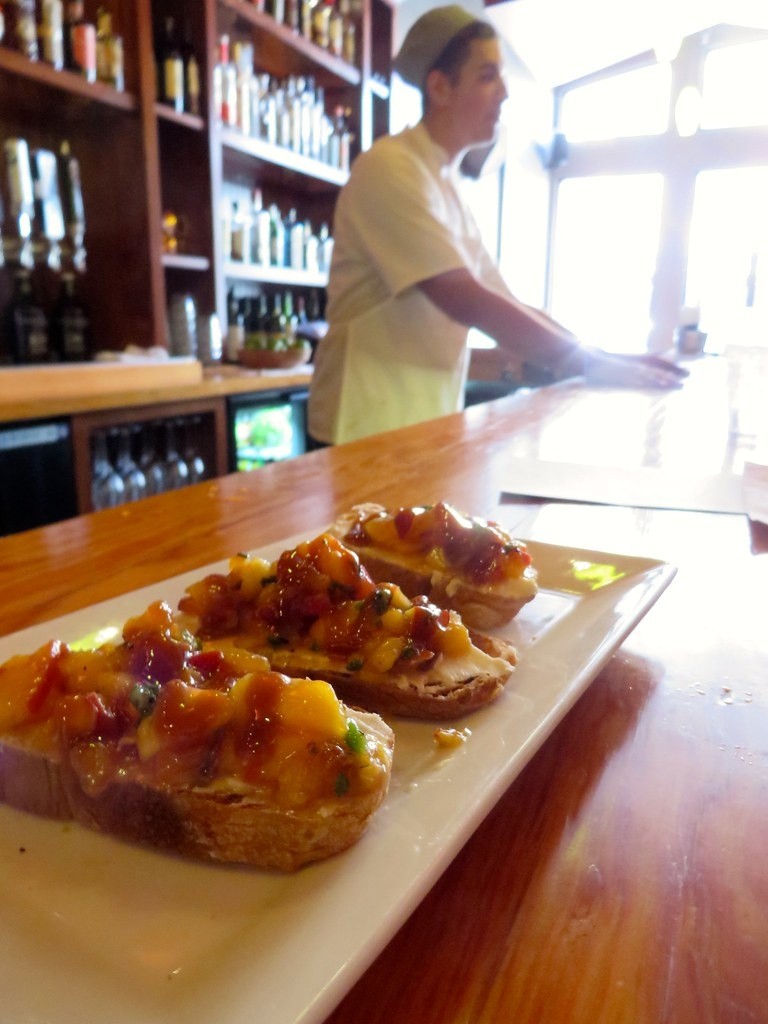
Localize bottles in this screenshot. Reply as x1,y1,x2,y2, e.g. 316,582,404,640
0,137,88,364
231,188,333,270
1,0,123,90
224,287,320,364
250,0,356,61
156,16,200,118
217,32,349,174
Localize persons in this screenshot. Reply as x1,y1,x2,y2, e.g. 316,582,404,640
307,6,690,453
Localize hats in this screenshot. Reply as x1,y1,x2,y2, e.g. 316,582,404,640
394,6,474,88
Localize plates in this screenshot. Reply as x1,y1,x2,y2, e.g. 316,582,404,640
1,524,678,1024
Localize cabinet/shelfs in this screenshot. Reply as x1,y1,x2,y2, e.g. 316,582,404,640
0,0,396,536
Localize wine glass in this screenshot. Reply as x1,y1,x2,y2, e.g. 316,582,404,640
88,416,206,509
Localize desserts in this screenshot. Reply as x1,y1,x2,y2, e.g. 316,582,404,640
166,531,519,723
324,502,540,631
0,599,398,874
